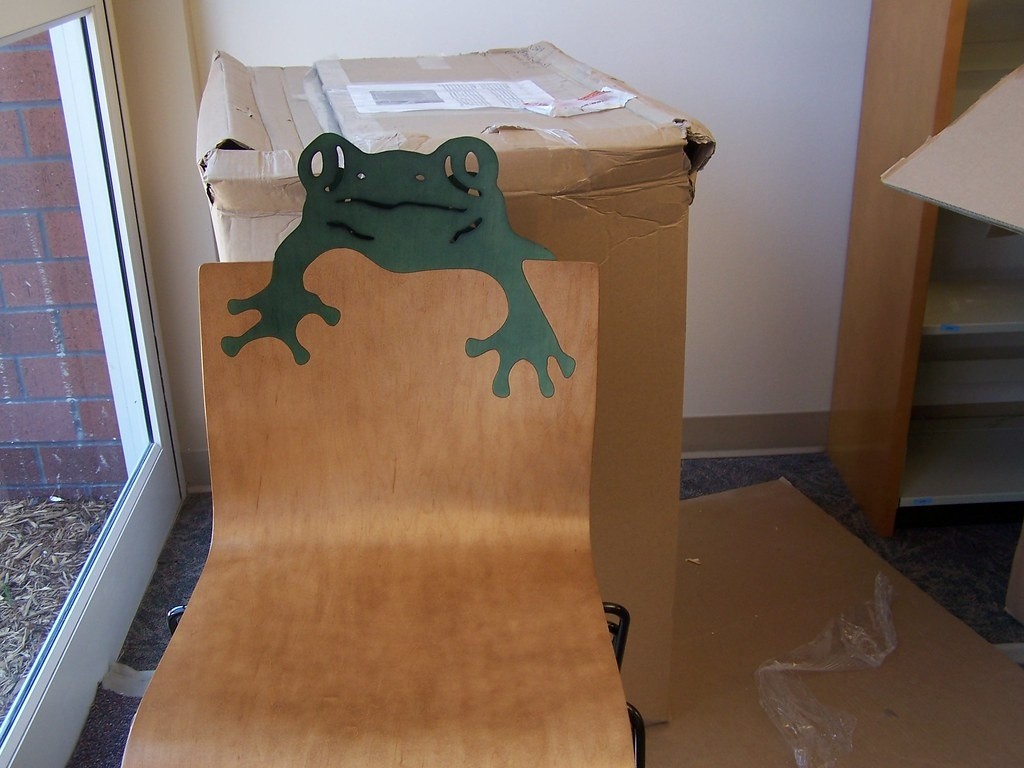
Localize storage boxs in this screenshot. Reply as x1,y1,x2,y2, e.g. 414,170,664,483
194,39,716,725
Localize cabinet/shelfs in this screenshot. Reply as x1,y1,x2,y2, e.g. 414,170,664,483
826,0,1024,530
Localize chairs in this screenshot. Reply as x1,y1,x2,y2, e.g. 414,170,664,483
119,260,642,768
